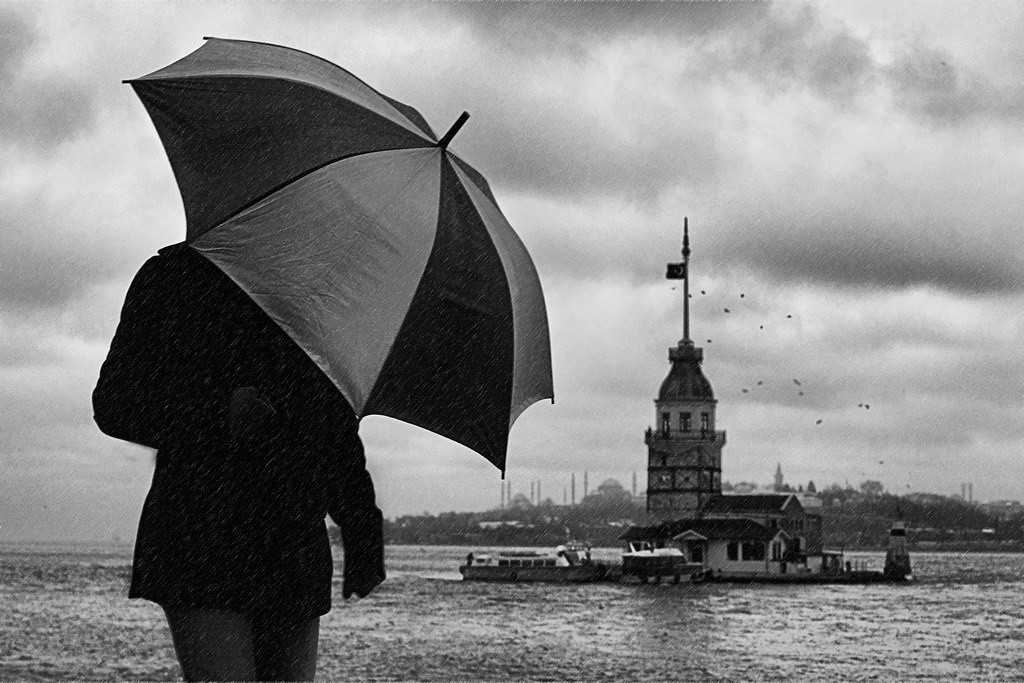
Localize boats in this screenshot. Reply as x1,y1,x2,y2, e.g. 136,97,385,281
617,548,706,585
458,545,601,583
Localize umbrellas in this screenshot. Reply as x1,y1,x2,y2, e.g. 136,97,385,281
119,31,556,482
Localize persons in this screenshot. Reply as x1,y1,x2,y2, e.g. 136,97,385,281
584,541,596,562
466,551,473,566
92,237,389,683
